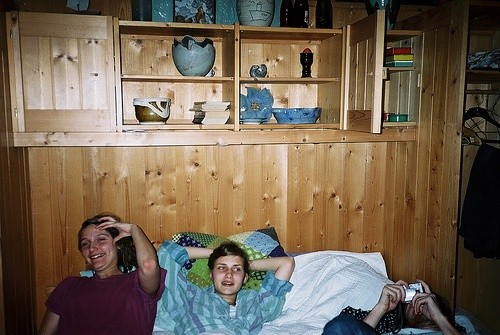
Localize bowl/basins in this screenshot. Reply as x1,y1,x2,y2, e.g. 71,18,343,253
133,97,171,125
271,107,322,124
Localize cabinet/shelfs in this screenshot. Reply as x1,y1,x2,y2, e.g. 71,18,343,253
5,0,500,133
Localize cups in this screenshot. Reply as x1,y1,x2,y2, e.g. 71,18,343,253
300,52,314,78
131,0,152,21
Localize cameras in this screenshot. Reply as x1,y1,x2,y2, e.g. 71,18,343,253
404,283,424,304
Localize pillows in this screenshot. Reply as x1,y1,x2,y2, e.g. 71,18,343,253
172,227,287,257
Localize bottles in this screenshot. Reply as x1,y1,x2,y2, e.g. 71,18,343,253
365,0,401,29
279,0,333,29
236,0,275,27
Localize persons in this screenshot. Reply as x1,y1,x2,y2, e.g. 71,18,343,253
38,211,167,335
156,240,295,335
321,278,466,335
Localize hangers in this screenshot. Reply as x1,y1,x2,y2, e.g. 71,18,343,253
463,89,500,146
